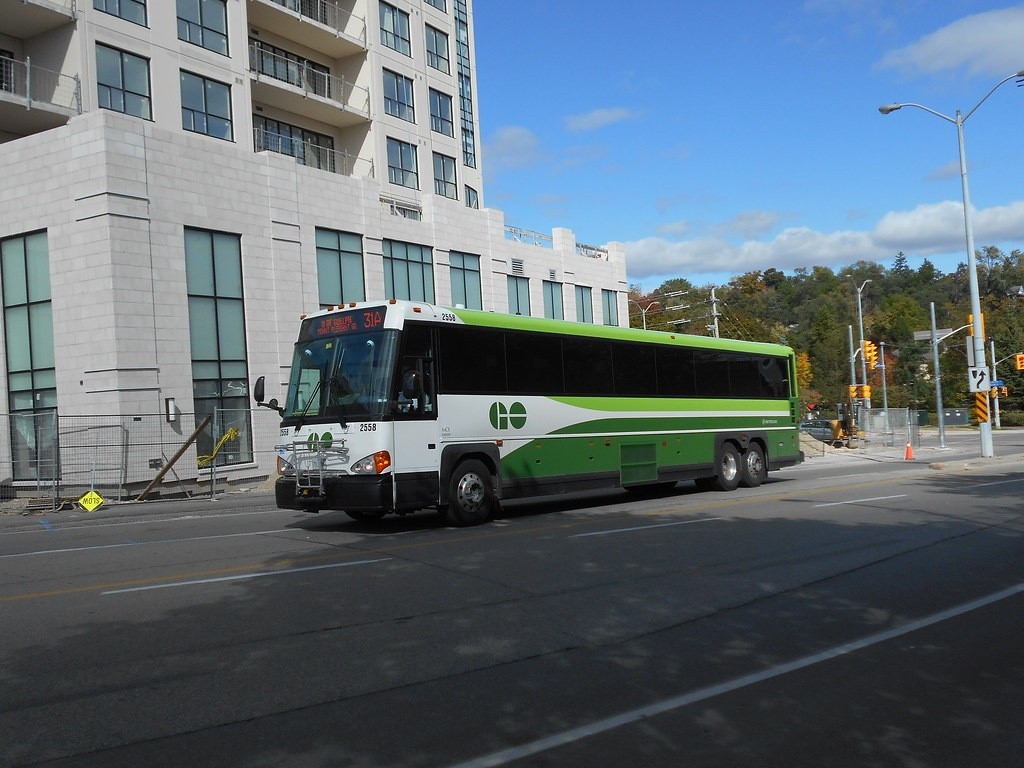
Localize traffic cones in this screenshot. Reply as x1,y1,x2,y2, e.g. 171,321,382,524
904,442,915,461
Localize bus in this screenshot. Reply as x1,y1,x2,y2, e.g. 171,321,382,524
254,298,805,526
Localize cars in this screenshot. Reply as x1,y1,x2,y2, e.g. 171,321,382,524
800,419,836,440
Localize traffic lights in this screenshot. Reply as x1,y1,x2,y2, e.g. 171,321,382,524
849,387,858,400
1016,354,1024,370
870,351,879,369
864,341,873,360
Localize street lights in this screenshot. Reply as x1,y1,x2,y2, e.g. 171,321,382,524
931,300,975,450
857,271,886,409
628,299,660,330
878,70,1017,458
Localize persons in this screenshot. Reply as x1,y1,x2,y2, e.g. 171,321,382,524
334,368,430,413
